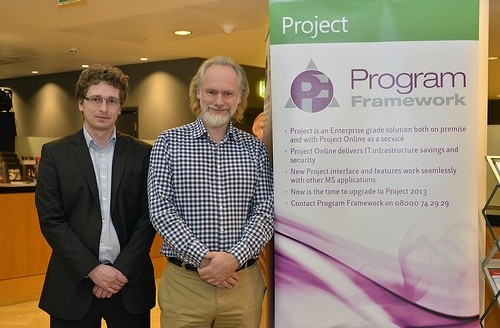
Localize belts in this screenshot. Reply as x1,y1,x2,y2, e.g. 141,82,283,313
169,257,256,271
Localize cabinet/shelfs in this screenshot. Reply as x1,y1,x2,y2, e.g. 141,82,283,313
480,155,500,328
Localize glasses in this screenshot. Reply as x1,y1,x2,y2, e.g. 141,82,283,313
83,96,120,107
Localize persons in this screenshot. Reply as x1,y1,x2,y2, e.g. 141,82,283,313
34,63,157,328
252,112,263,140
146,55,275,328
